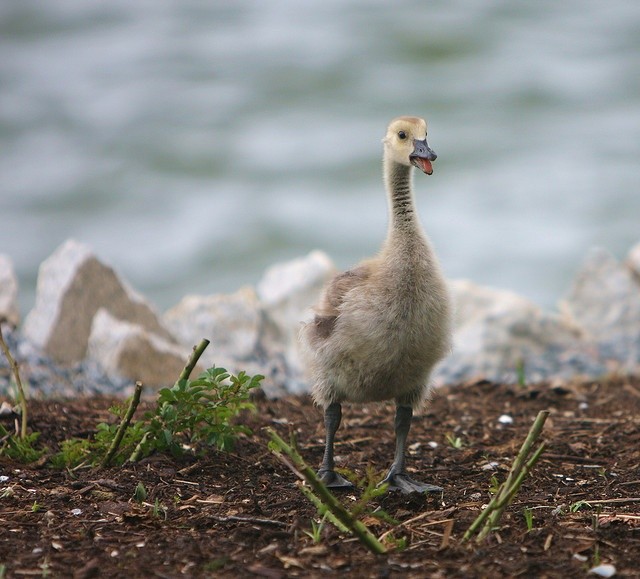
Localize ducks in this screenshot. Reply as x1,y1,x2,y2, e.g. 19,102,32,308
299,117,457,496
